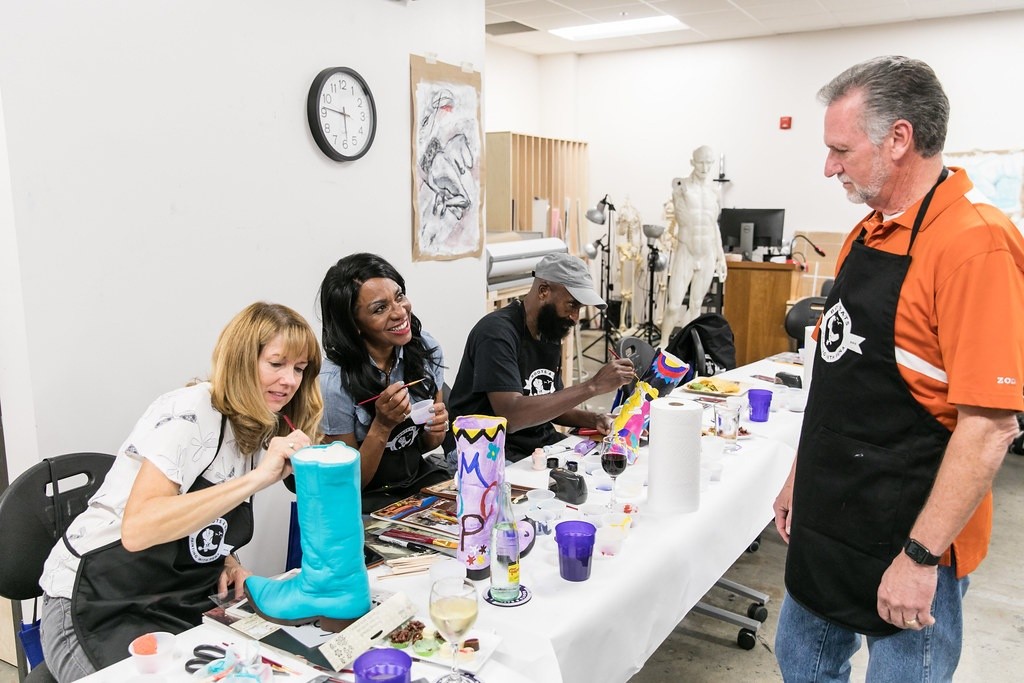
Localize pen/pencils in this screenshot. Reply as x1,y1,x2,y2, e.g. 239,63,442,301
379,536,427,554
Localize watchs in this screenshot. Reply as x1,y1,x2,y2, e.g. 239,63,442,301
903,537,941,566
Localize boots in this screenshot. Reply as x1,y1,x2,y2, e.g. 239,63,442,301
450,415,537,578
242,442,371,631
609,347,691,465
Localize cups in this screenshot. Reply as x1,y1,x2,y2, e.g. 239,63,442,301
726,383,805,422
799,349,805,362
353,648,412,682
511,452,645,556
554,520,596,582
713,401,742,451
128,631,177,674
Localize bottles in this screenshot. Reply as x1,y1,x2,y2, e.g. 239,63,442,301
489,482,520,602
531,447,547,471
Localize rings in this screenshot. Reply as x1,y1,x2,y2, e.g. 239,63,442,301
290,443,294,448
903,620,917,626
403,413,407,417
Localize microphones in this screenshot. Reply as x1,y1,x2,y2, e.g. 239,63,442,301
787,235,826,273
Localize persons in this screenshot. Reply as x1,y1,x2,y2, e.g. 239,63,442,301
35,303,323,681
444,253,636,467
773,58,1024,683
660,146,727,355
320,252,452,514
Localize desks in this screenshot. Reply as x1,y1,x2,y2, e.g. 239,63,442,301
69,350,814,683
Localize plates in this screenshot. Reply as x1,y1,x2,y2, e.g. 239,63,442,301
681,376,755,397
703,423,753,440
380,616,502,675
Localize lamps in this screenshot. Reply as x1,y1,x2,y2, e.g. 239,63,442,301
573,192,624,364
583,232,619,331
631,223,662,348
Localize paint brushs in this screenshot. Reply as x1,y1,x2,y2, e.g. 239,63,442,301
282,414,297,432
432,511,458,524
607,347,641,383
222,641,297,674
357,377,427,407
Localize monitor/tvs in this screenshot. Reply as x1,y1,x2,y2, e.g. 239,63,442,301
720,208,785,262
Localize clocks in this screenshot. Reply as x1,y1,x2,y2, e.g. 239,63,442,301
307,66,377,162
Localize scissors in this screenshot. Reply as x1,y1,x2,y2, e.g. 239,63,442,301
186,644,290,677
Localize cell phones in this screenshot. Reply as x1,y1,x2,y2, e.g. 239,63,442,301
363,544,384,566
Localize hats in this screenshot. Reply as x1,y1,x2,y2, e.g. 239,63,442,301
530,252,608,310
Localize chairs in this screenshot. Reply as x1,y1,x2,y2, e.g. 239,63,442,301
618,337,675,397
0,451,118,683
785,297,828,353
690,328,708,379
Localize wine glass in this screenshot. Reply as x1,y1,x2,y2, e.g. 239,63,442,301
601,435,628,511
429,577,480,683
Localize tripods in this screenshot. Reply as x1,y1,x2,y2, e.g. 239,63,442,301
573,202,661,365
